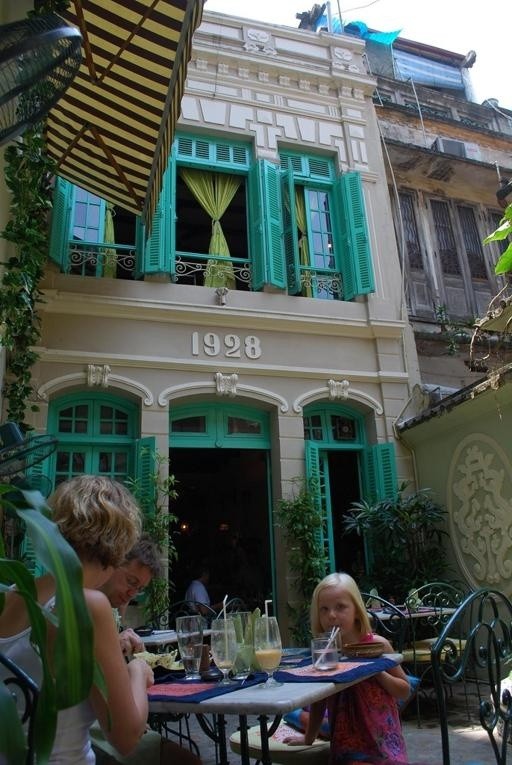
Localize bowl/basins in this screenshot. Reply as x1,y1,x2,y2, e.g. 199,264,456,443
344,642,384,658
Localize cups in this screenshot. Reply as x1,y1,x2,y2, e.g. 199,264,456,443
311,633,343,672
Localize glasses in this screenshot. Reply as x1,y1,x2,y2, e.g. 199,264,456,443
121,567,146,596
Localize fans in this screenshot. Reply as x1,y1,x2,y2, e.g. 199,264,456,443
2,422,60,520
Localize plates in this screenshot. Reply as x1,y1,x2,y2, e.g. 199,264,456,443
134,628,153,636
153,666,186,684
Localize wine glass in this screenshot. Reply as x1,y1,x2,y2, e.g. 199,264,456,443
175,613,284,688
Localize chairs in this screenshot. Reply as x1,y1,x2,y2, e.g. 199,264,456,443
0,578,511,765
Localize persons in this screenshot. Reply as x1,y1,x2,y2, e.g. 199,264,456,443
89,532,203,765
282,572,410,765
185,565,223,628
0,475,155,765
